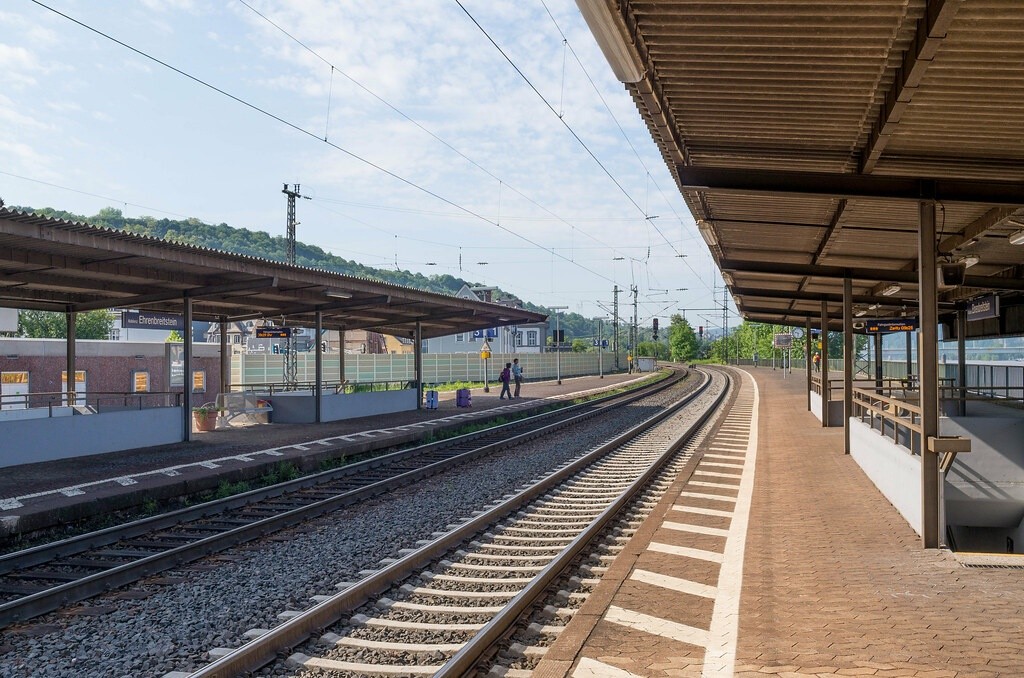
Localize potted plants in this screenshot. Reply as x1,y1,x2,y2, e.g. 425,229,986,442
193,406,226,431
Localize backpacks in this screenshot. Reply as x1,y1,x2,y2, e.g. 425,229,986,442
499,368,506,382
813,356,817,362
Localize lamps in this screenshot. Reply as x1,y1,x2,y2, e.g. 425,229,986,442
1007,228,1024,246
321,289,352,299
733,295,742,306
882,283,903,296
855,308,867,318
954,254,979,268
575,0,646,84
722,272,736,287
869,303,882,310
696,220,719,246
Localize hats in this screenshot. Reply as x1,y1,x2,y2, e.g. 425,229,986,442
815,352,818,354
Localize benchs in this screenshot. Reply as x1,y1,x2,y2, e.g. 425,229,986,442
217,391,273,428
867,391,917,418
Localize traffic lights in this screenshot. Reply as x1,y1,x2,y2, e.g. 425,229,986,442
321,341,326,352
274,343,280,354
698,326,703,334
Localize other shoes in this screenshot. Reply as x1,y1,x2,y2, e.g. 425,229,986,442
510,397,515,400
500,396,505,399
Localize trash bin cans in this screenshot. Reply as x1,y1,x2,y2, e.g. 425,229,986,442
410,380,423,405
255,399,273,423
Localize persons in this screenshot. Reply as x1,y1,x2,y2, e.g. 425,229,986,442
813,352,820,373
499,363,514,400
512,358,522,399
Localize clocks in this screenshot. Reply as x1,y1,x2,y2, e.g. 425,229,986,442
792,328,804,339
